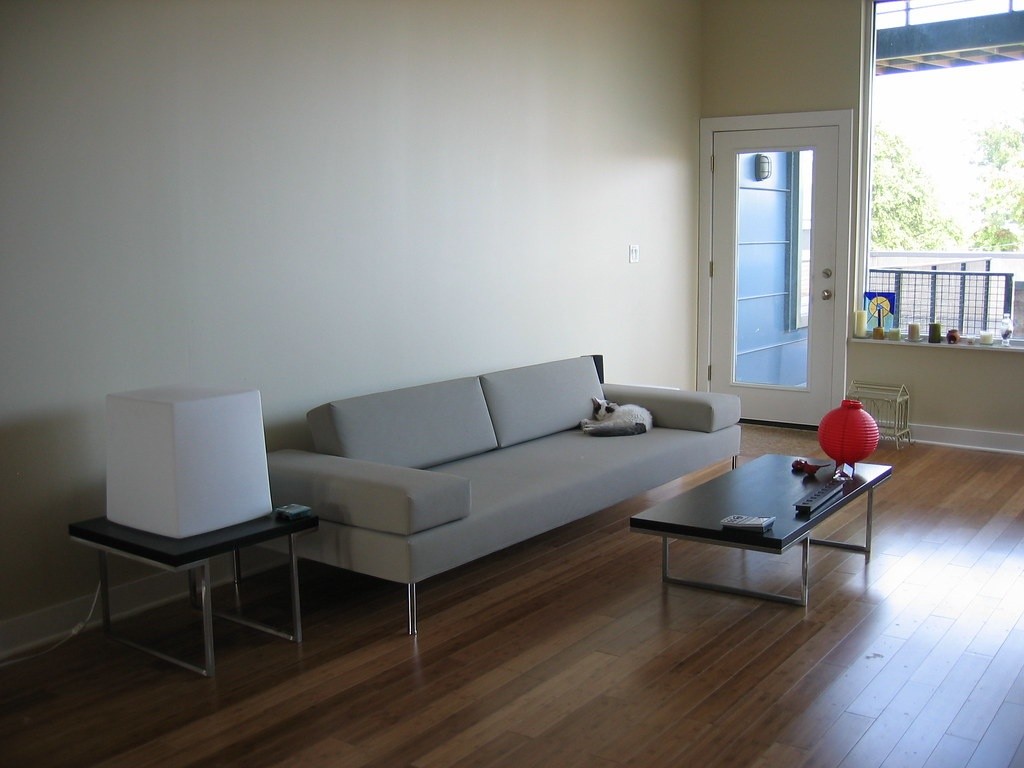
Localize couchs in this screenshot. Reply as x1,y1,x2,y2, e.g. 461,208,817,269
264,354,741,637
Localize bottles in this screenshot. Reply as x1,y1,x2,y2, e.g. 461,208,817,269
1001,313,1013,346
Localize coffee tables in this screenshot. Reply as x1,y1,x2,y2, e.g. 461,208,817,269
629,453,893,607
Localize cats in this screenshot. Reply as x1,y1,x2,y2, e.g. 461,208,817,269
579,397,653,437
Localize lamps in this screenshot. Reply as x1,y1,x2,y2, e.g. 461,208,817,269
105,384,273,541
755,153,773,181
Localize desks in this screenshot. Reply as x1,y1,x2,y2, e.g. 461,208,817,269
68,506,321,681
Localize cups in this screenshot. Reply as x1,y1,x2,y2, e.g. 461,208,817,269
979,330,994,345
929,324,942,343
857,311,867,336
873,328,883,339
908,324,920,340
889,328,902,340
